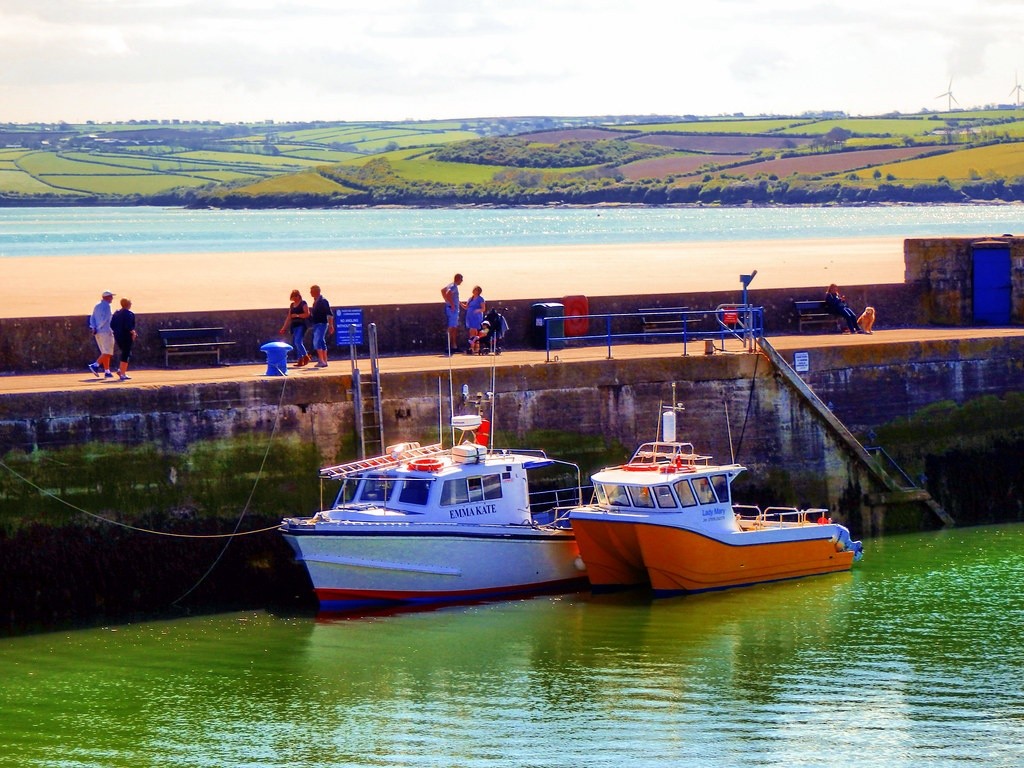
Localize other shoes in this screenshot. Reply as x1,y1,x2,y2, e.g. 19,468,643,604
116,369,121,375
121,376,132,381
105,372,114,378
452,347,464,353
468,339,474,344
445,349,453,353
89,363,100,377
857,330,863,333
314,361,328,367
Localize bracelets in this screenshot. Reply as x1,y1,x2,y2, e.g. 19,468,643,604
297,315,299,317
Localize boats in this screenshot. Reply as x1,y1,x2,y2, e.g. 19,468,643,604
277,417,596,614
568,382,864,599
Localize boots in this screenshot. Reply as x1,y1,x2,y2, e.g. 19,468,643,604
303,353,311,365
293,356,305,367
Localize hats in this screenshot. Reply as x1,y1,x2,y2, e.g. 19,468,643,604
481,321,491,326
102,291,117,298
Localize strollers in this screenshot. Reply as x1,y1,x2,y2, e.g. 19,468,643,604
466,306,507,356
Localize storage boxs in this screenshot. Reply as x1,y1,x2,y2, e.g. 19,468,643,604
451,444,488,465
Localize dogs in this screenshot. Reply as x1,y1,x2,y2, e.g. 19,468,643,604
856,307,875,333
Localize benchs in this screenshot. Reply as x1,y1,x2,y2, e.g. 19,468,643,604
159,327,238,369
794,301,842,333
638,307,703,343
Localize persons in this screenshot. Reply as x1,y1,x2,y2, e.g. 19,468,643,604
281,289,311,367
310,284,334,367
824,284,864,334
468,320,490,344
442,273,463,355
460,285,486,352
110,297,138,380
88,291,117,378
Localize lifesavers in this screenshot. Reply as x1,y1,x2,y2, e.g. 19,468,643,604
623,463,659,471
660,465,696,473
409,458,444,471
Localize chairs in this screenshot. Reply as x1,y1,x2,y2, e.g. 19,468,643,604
659,495,676,507
614,494,629,505
